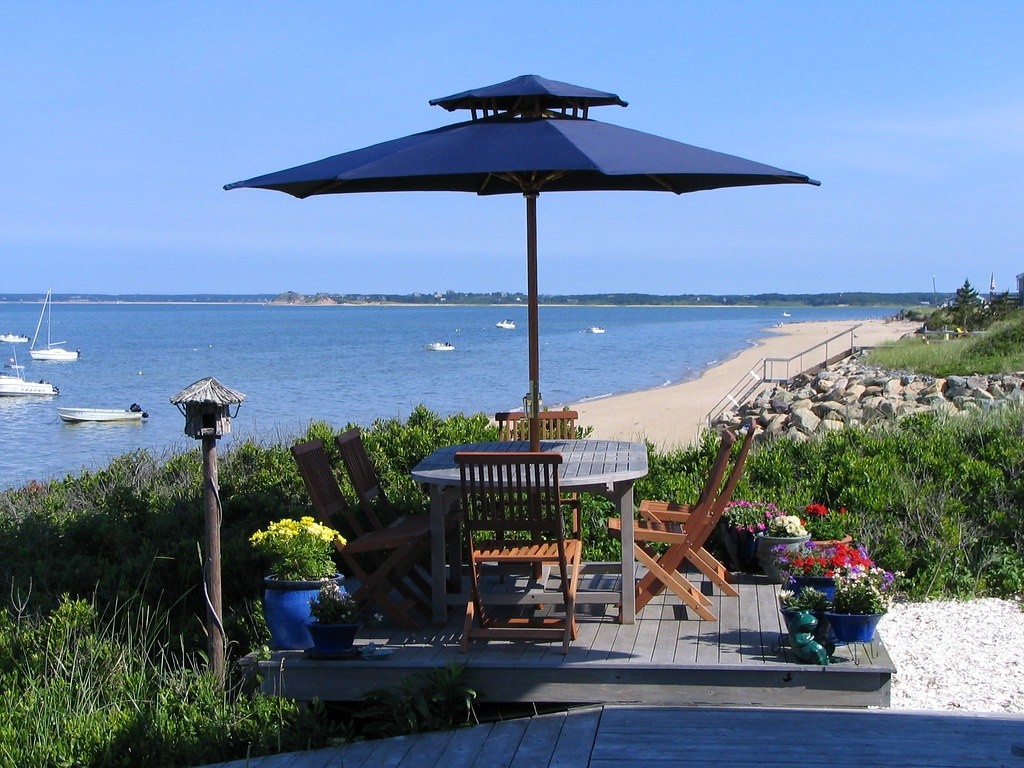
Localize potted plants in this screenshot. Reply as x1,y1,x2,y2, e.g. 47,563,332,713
308,584,366,658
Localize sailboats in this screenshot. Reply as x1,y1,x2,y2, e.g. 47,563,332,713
29,285,81,361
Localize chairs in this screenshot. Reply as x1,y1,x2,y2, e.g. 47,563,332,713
290,412,756,655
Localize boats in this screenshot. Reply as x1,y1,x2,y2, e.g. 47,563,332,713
0,346,61,397
496,318,516,329
586,326,605,333
423,341,455,351
0,332,31,344
57,403,149,423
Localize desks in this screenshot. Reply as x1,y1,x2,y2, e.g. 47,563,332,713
411,440,649,625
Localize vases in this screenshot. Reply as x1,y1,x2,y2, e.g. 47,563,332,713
814,535,854,549
782,577,840,639
759,530,809,584
832,607,888,642
784,604,833,659
264,572,350,650
722,516,755,573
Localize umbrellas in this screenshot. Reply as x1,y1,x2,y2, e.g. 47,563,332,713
220,75,820,451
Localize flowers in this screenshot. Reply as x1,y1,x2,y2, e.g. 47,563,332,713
722,495,903,615
250,517,350,580
